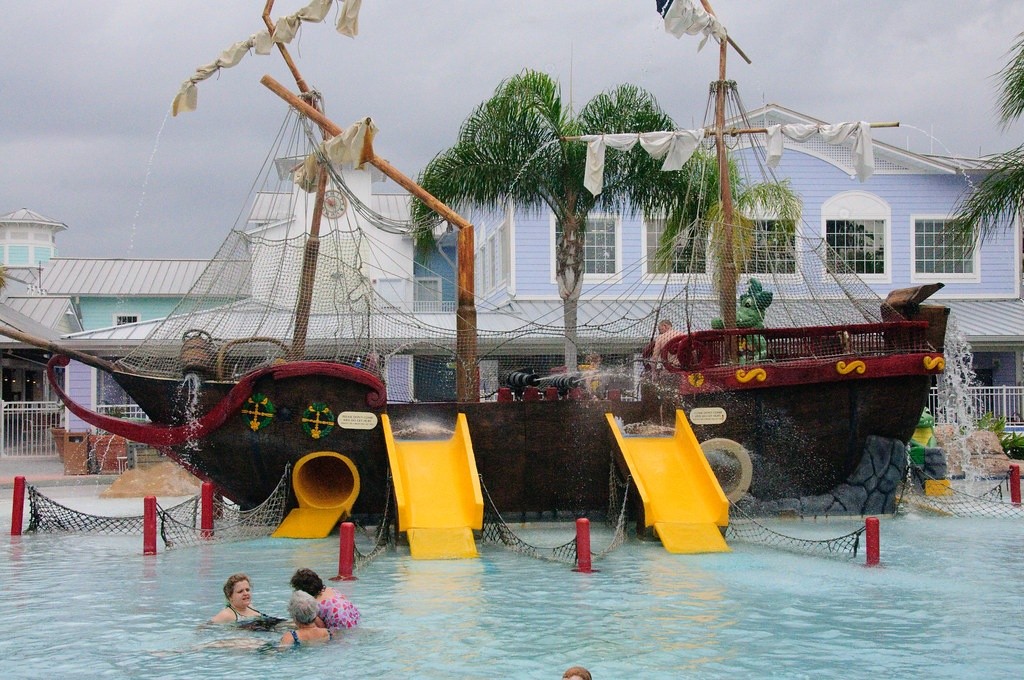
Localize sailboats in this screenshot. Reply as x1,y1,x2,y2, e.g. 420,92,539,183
0,0,954,525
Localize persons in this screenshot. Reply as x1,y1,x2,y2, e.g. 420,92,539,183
652,319,687,383
211,569,360,646
562,667,592,680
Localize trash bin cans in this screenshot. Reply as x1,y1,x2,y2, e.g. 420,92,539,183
64,432,89,475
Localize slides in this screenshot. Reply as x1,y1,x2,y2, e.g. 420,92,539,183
605,410,729,554
381,413,484,560
